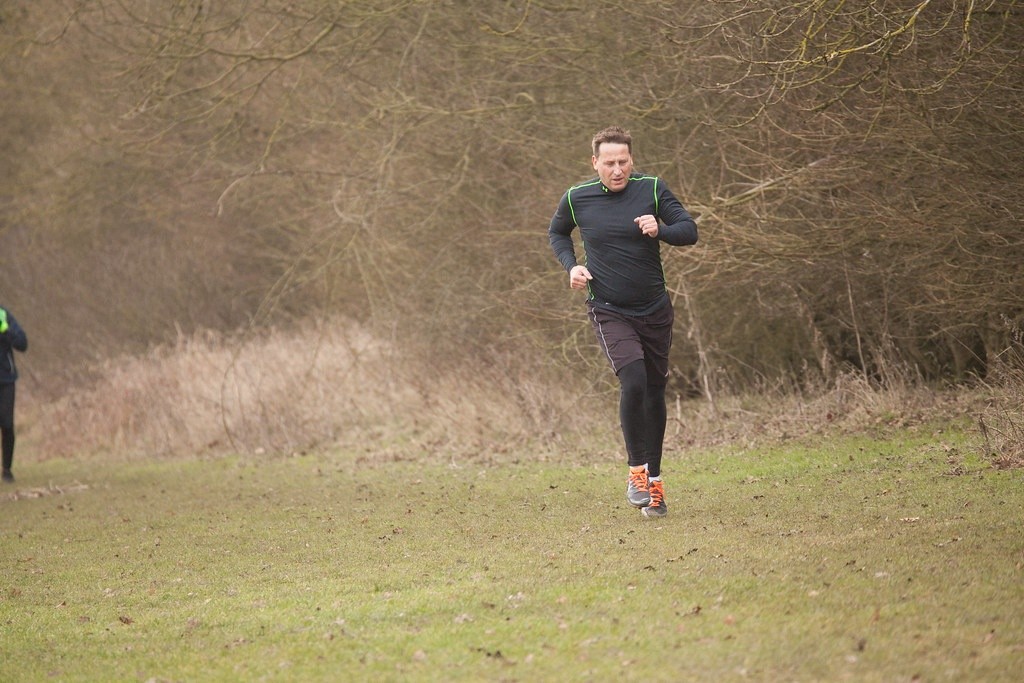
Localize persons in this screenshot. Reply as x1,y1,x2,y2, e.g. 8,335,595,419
0,306,27,485
546,127,697,520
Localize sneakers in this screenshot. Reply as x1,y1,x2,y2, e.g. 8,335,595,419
641,479,666,518
626,465,651,508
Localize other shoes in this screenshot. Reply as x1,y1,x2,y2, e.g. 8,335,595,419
3,470,15,483
